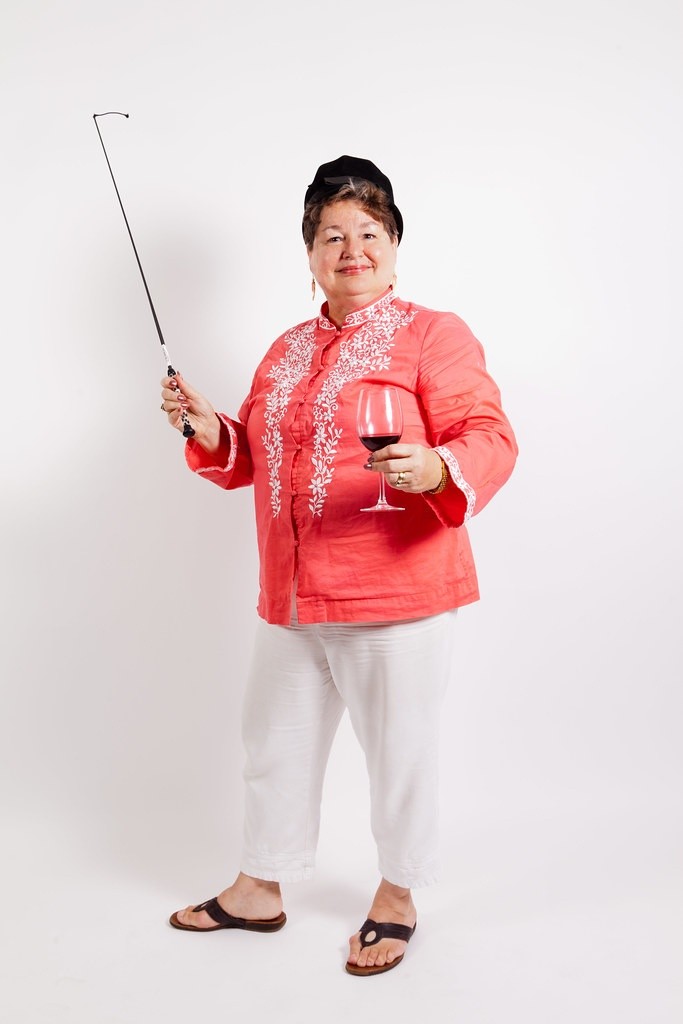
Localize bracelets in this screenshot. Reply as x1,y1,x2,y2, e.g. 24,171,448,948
428,459,449,494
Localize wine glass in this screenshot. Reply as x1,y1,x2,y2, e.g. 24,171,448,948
356,386,405,512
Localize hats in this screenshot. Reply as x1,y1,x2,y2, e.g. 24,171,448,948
304,155,403,245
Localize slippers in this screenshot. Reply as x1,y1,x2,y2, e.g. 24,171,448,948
170,897,288,933
345,912,417,975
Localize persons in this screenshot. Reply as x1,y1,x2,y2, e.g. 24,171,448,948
159,156,518,976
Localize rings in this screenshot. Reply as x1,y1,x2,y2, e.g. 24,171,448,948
397,472,403,483
161,404,166,411
